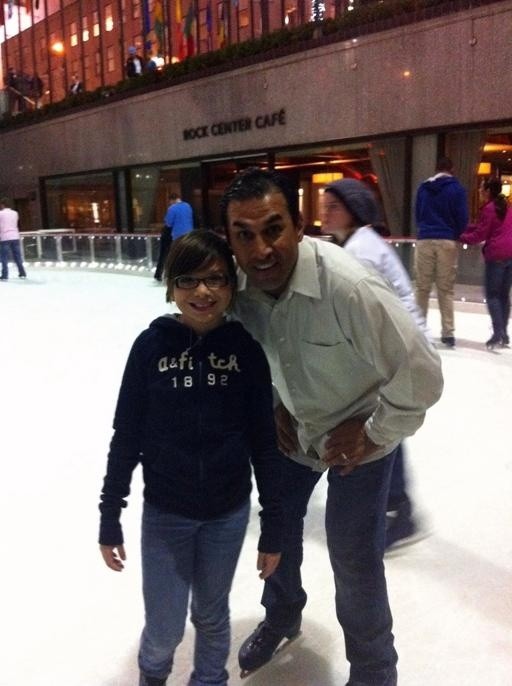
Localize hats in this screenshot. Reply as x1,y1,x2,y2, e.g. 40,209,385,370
324,178,381,225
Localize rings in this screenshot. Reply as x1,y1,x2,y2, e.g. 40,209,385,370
337,449,349,463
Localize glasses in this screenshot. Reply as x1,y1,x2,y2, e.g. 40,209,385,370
172,271,233,290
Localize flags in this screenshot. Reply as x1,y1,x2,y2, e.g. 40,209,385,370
141,0,240,61
1,0,41,29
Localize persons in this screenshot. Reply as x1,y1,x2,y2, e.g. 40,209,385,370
5,66,44,116
126,45,146,80
314,173,432,547
67,74,84,96
1,197,27,281
143,47,158,74
218,165,447,686
162,191,193,243
98,229,285,686
411,154,468,343
459,176,512,347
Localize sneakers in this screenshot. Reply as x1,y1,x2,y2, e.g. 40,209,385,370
385,498,411,532
441,337,454,345
345,668,398,685
485,335,510,345
238,611,300,670
139,672,167,685
0,274,26,279
